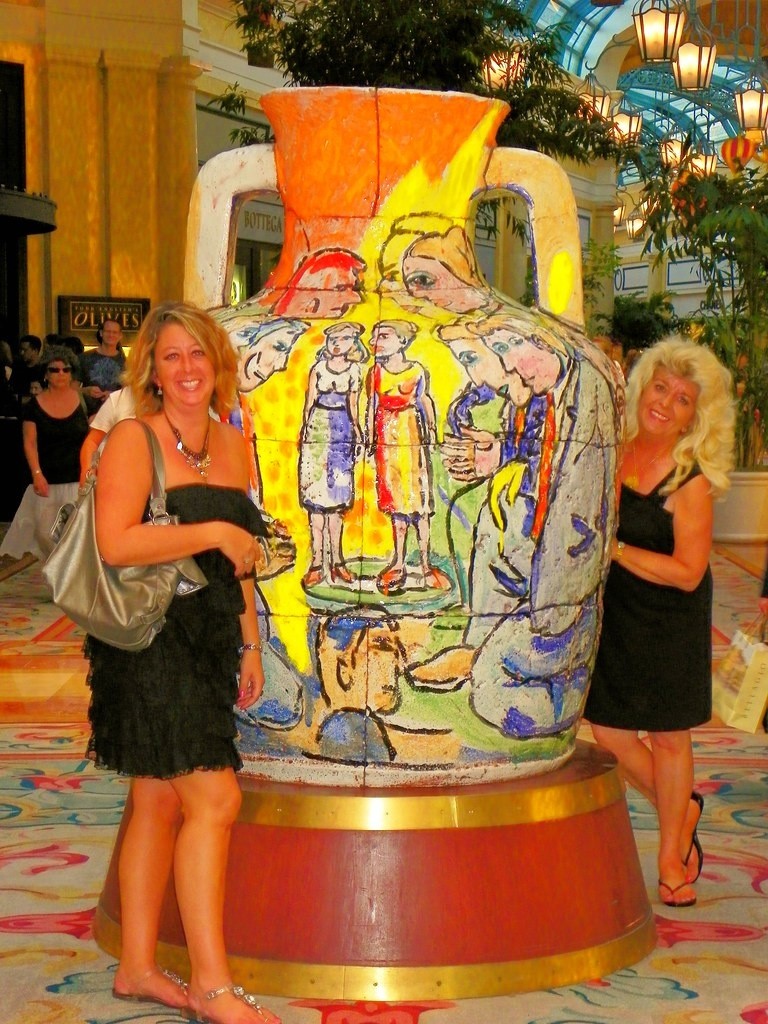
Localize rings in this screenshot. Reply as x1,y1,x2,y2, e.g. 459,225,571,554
244,560,249,563
36,491,40,494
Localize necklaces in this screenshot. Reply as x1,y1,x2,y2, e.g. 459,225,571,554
626,436,669,491
164,410,212,480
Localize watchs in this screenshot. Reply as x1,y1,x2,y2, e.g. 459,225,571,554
614,541,625,561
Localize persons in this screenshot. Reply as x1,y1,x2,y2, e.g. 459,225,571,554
584,335,736,905
81,301,283,1024
0,320,160,522
590,334,645,375
759,557,768,735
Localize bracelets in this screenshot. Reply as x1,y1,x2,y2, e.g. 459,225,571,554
32,469,42,474
242,644,262,651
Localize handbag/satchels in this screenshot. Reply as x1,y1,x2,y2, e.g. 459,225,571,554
712,613,768,734
40,416,208,652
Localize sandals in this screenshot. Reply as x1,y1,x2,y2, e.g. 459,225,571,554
181,982,281,1024
112,963,189,1009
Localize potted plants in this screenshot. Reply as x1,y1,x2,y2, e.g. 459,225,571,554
642,133,768,543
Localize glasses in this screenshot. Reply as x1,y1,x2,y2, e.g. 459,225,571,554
46,367,71,373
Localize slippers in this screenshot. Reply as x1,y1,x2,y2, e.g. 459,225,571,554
658,878,696,907
682,791,703,884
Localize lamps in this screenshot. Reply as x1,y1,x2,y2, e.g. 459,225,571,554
630,0,688,63
669,6,718,92
609,73,642,149
661,115,718,180
575,44,636,119
733,65,768,161
611,187,660,241
481,27,531,91
